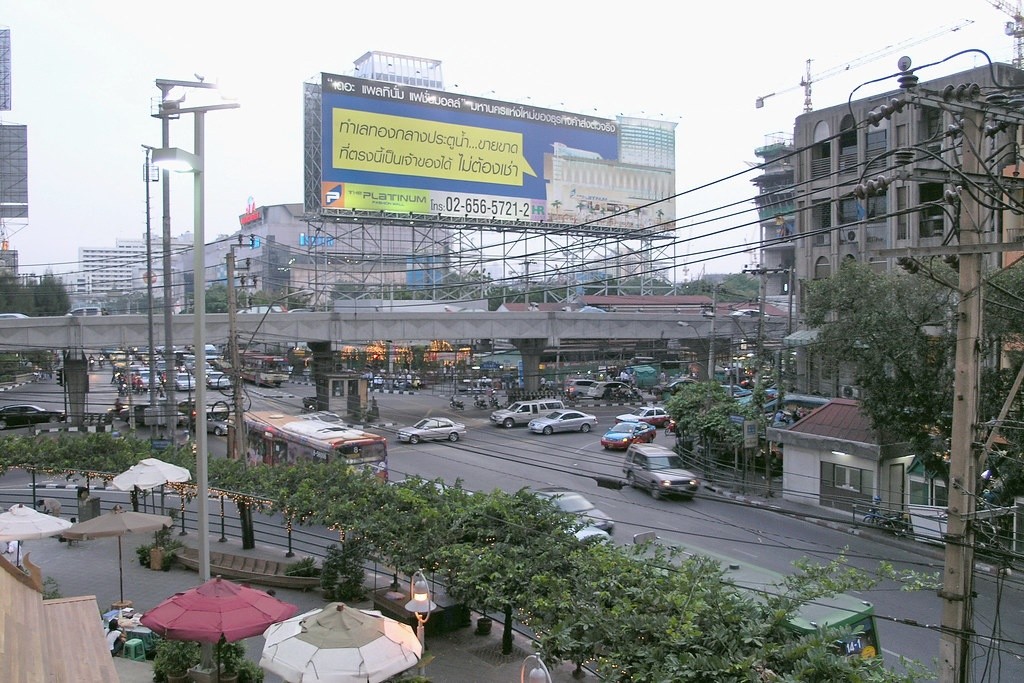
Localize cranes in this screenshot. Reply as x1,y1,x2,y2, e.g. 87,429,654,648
986,0,1024,68
754,15,977,114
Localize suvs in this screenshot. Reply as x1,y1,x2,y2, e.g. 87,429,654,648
623,444,699,500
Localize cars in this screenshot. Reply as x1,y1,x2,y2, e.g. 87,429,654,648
571,306,606,313
397,417,467,443
568,376,830,454
533,405,672,532
0,305,232,438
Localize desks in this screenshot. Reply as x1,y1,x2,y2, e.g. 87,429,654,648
103,617,153,650
111,600,133,610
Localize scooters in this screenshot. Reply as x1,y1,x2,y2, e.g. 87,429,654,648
867,503,908,536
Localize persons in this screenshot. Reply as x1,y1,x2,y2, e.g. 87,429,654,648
115,398,125,414
606,370,635,388
5,541,22,566
266,590,276,598
658,371,666,382
59,518,76,547
36,498,61,518
540,376,546,389
97,351,105,365
161,371,166,386
111,370,143,394
104,618,126,657
88,354,94,367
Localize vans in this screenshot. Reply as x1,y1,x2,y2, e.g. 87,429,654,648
491,400,566,426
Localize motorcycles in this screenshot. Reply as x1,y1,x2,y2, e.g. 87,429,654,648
448,395,500,410
665,418,678,437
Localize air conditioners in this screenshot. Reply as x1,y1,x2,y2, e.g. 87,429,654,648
840,384,858,399
846,230,859,243
817,234,830,245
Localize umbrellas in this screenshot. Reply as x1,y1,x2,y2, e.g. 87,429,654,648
259,602,423,683
139,575,299,683
113,458,192,548
62,505,172,604
0,503,73,567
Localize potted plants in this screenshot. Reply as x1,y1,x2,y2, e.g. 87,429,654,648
165,649,189,683
320,563,339,602
476,603,497,632
220,662,239,683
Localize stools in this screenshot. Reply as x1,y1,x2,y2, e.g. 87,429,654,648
123,638,146,662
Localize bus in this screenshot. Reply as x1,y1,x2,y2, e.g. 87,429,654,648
229,350,289,387
241,411,388,486
616,530,884,680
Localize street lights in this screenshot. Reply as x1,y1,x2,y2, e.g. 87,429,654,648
403,567,436,680
676,321,714,407
144,70,238,670
